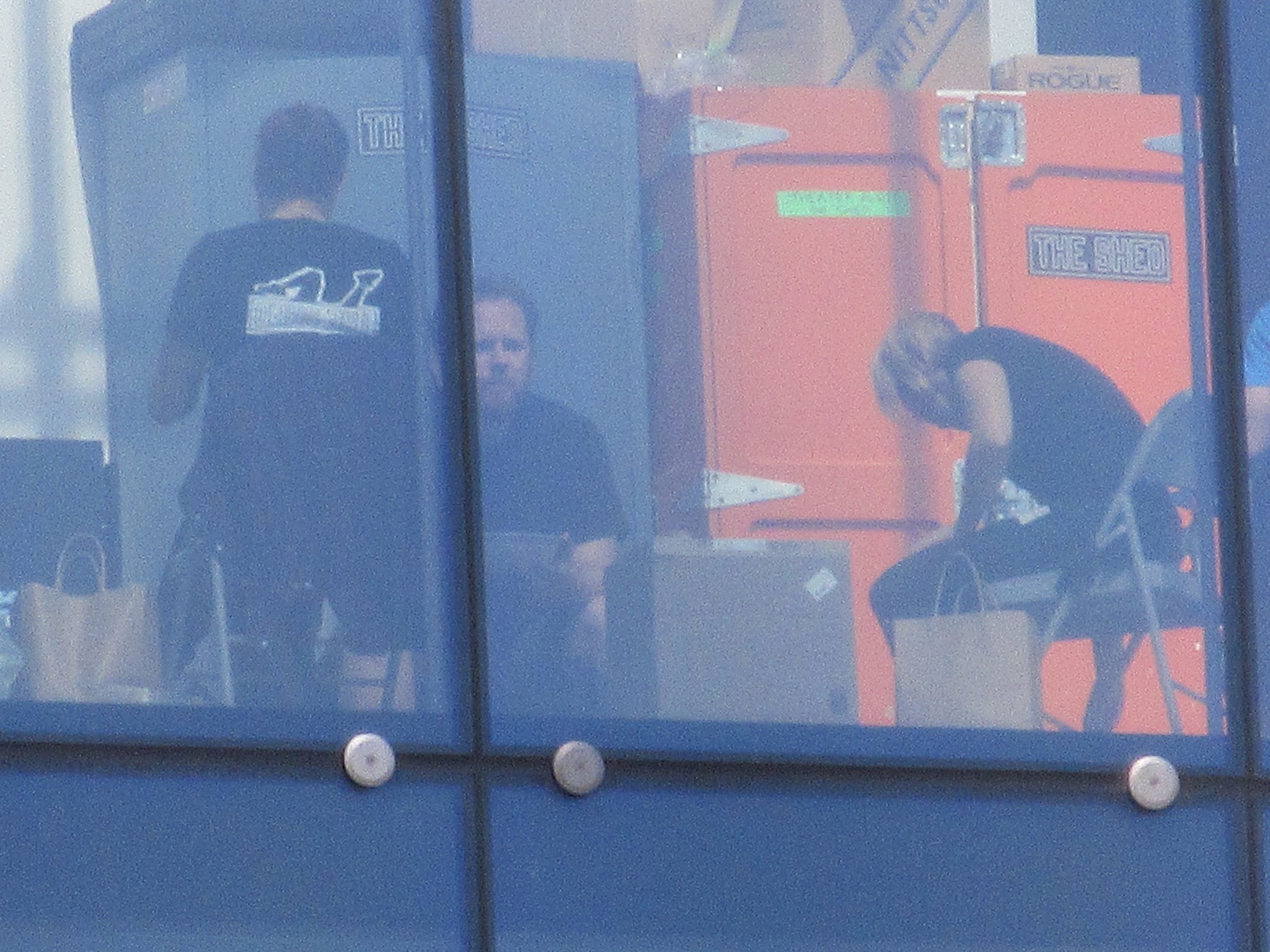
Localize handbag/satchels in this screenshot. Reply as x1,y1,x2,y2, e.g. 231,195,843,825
18,531,166,708
890,550,1043,730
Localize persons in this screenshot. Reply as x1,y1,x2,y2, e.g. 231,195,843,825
871,311,1180,731
147,106,414,707
1240,298,1270,742
471,271,628,679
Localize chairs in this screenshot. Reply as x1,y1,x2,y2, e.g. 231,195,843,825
978,390,1228,735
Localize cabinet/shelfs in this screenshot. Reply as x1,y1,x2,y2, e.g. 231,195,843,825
605,537,858,725
640,82,1227,734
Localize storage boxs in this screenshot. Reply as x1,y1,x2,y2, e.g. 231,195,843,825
990,54,1142,94
